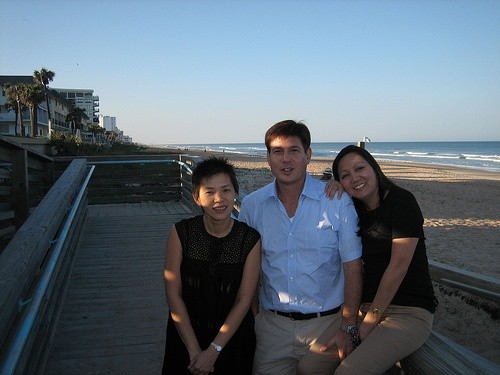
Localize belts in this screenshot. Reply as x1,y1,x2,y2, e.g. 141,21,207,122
268,305,342,320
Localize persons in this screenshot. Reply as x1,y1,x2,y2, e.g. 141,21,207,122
162,156,261,375
298,145,438,374
236,120,362,374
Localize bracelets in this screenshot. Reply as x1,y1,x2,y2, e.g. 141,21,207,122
341,323,362,351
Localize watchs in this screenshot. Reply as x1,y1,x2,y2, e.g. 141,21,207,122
370,305,383,316
211,342,222,352
